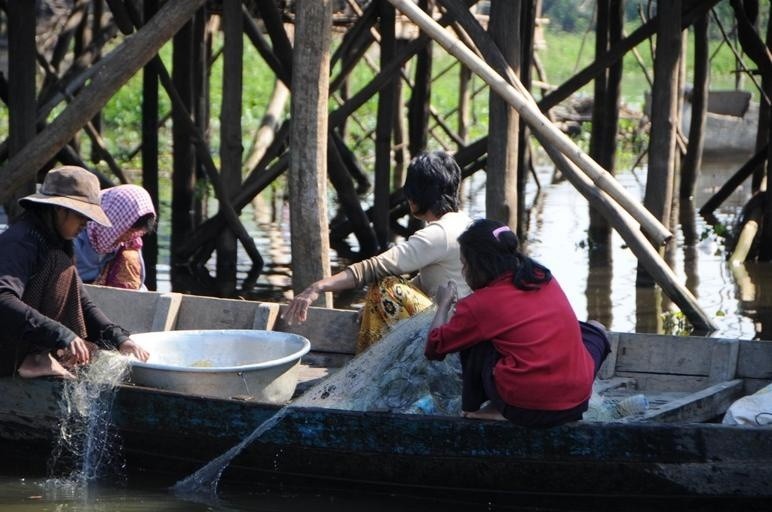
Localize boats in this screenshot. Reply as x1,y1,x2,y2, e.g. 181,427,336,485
645,90,760,155
0,283,772,512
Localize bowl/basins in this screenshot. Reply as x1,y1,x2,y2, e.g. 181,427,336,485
119,330,312,404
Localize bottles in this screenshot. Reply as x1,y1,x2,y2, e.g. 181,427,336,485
608,395,650,420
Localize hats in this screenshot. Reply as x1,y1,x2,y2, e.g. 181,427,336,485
16,165,113,230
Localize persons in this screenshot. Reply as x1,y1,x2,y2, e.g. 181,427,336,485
1,165,152,380
279,149,476,366
73,183,158,291
423,218,612,430
